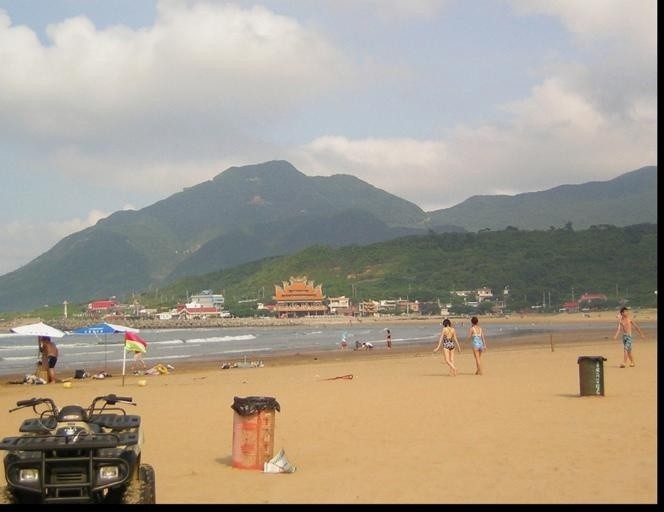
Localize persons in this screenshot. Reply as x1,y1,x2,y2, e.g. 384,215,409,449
362,342,373,351
130,350,145,370
35,336,58,384
463,316,487,375
432,319,461,376
614,306,645,368
386,329,391,351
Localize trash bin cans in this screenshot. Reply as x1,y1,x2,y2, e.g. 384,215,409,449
231,396,280,470
577,356,607,396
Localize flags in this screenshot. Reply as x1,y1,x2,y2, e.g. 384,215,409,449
124,332,146,353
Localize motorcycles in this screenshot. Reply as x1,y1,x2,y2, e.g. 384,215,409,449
0,394,155,504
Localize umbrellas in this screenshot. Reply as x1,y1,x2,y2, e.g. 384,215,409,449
10,321,64,364
64,322,140,371
